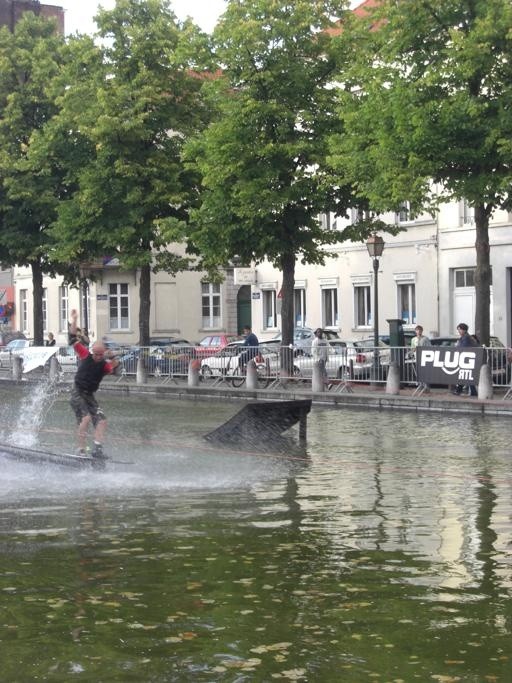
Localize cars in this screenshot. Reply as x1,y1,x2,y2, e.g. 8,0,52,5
199,342,280,383
291,339,388,382
56,340,118,375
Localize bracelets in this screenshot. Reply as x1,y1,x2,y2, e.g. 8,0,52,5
108,355,116,359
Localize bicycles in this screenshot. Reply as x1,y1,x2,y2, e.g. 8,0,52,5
231,359,270,389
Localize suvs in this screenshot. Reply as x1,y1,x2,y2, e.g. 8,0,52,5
275,328,343,351
194,332,245,365
368,329,425,348
0,338,46,370
118,337,194,375
405,336,511,387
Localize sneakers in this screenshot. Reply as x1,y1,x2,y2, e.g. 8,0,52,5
92,444,109,459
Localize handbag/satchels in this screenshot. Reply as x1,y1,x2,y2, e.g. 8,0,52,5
256,352,265,363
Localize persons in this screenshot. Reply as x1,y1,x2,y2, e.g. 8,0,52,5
67,309,120,460
45,331,56,347
456,323,478,396
452,334,480,395
77,327,90,353
312,327,336,391
233,324,258,373
411,325,434,362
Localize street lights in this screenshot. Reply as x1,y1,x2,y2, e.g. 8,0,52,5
366,231,385,384
78,260,92,341
